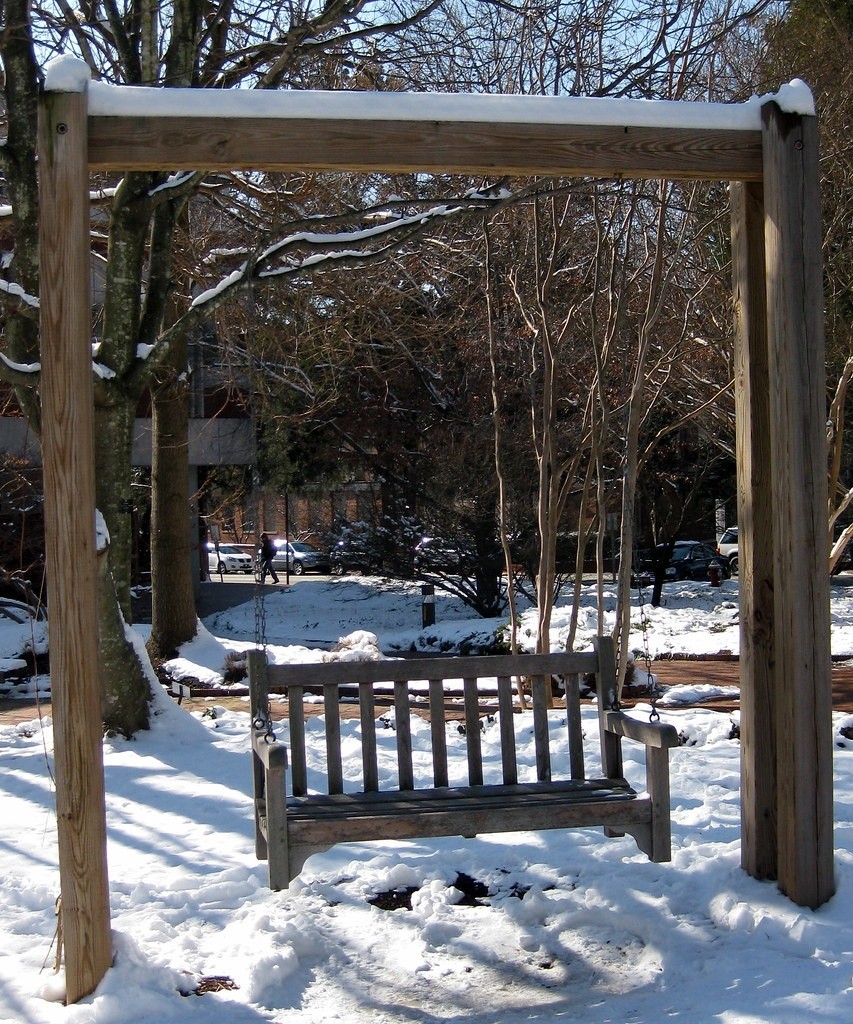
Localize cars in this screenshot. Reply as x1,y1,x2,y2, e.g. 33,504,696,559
269,541,329,577
205,543,253,577
334,525,741,591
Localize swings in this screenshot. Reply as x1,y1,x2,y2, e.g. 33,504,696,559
241,168,679,892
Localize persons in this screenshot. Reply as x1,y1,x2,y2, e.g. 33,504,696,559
255,533,279,584
